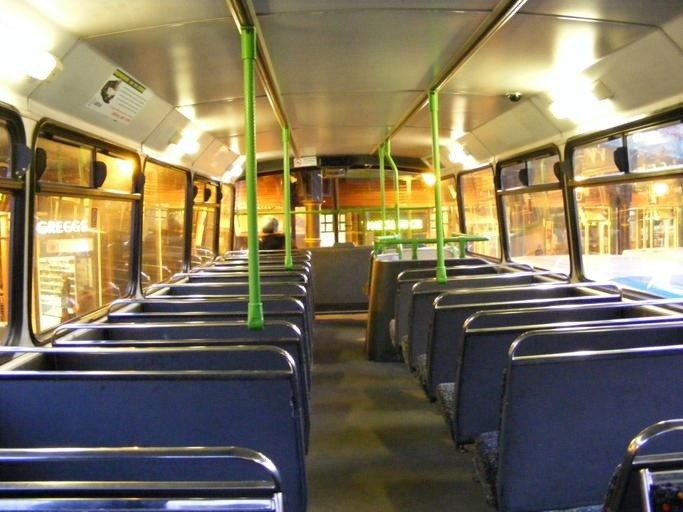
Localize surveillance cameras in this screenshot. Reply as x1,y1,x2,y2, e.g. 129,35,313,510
505,91,524,104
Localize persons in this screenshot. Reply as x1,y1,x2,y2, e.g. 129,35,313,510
261,216,278,233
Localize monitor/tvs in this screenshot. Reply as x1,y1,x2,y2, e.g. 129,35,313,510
305,175,332,197
